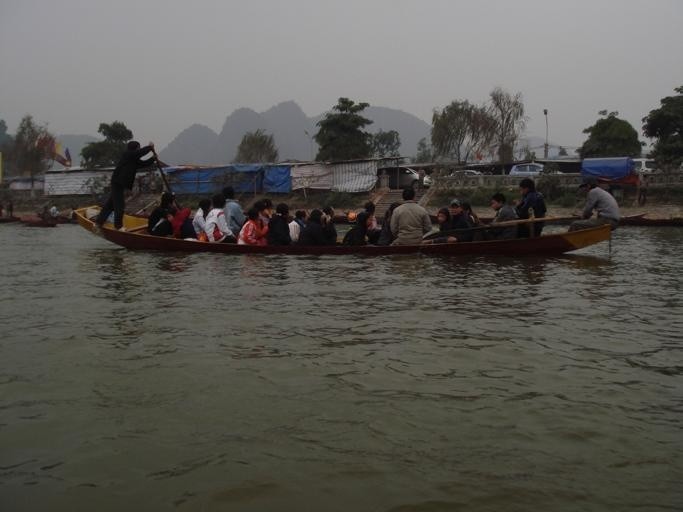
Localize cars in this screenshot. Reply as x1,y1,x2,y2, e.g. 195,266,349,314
445,169,482,176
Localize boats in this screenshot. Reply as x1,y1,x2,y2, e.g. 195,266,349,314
71,201,654,254
19,213,59,227
55,212,71,223
0,214,21,222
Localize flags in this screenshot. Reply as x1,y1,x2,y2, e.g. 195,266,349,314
35,137,71,169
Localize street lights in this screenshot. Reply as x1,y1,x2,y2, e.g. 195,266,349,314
542,107,549,160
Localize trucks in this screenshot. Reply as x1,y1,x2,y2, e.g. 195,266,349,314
581,157,657,176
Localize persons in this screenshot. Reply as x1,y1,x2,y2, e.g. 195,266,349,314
375,203,402,244
362,200,378,232
634,172,649,212
388,188,433,246
511,177,548,238
415,169,425,188
446,199,470,243
486,194,520,240
568,181,621,233
421,207,451,244
90,140,156,232
341,210,383,247
460,202,480,225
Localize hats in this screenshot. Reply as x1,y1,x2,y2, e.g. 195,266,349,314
449,198,461,208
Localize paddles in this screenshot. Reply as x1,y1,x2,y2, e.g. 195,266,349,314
422,212,650,239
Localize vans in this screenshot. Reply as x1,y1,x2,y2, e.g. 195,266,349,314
377,165,432,190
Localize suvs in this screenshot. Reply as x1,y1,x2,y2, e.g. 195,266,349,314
507,162,562,175
